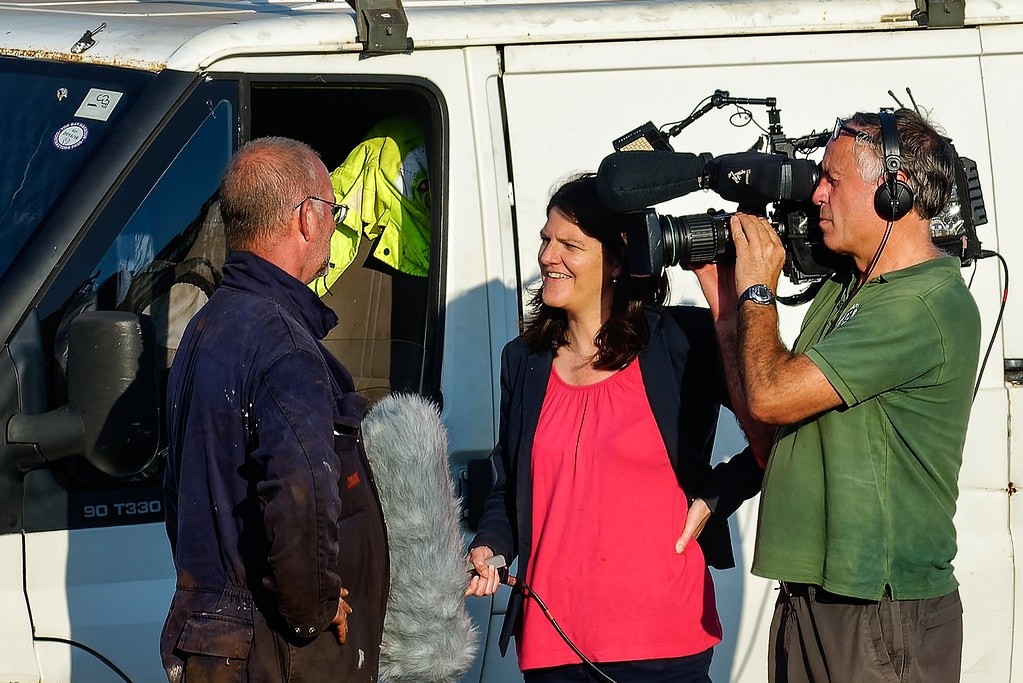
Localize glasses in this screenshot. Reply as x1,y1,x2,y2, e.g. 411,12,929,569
293,195,348,225
832,117,880,144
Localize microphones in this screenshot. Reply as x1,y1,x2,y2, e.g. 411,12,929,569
596,150,716,213
358,392,523,683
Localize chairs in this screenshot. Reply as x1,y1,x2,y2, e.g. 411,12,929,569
312,129,430,416
170,194,228,294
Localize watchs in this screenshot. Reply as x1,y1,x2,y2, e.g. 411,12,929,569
737,284,777,312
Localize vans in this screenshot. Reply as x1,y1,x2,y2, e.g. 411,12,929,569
0,0,1023,683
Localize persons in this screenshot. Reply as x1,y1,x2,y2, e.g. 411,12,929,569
157,137,391,683
690,109,982,683
463,174,764,683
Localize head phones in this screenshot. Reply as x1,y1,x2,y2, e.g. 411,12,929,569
874,112,914,223
